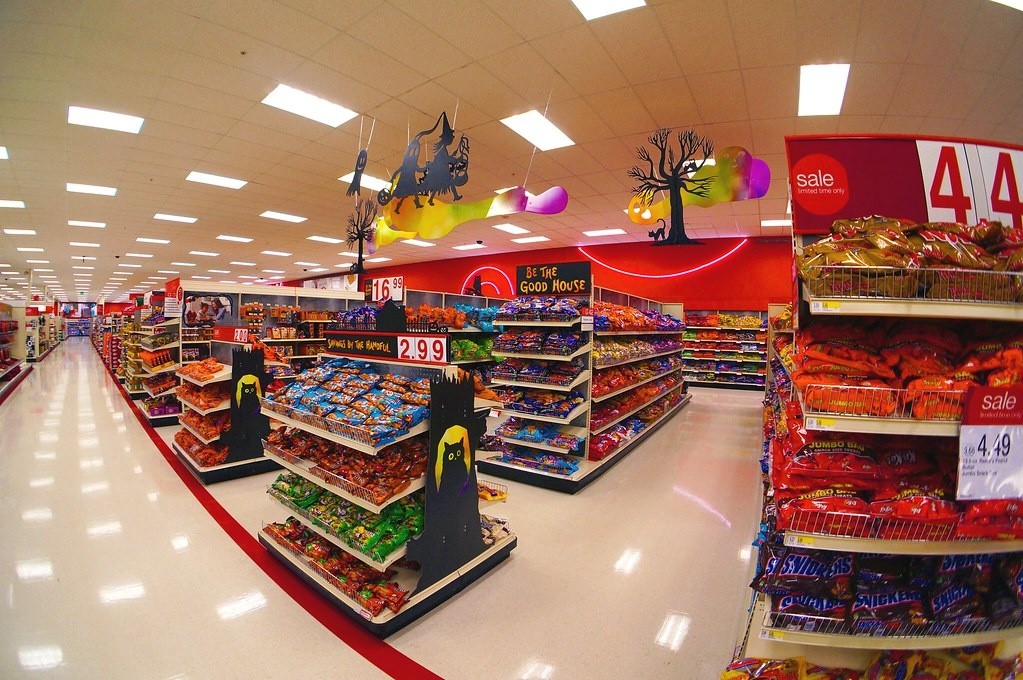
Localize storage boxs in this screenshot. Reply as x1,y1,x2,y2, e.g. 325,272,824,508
148,406,163,417
165,404,178,414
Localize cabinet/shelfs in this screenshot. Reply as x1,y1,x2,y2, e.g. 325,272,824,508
0,303,91,405
91,130,1023,679
